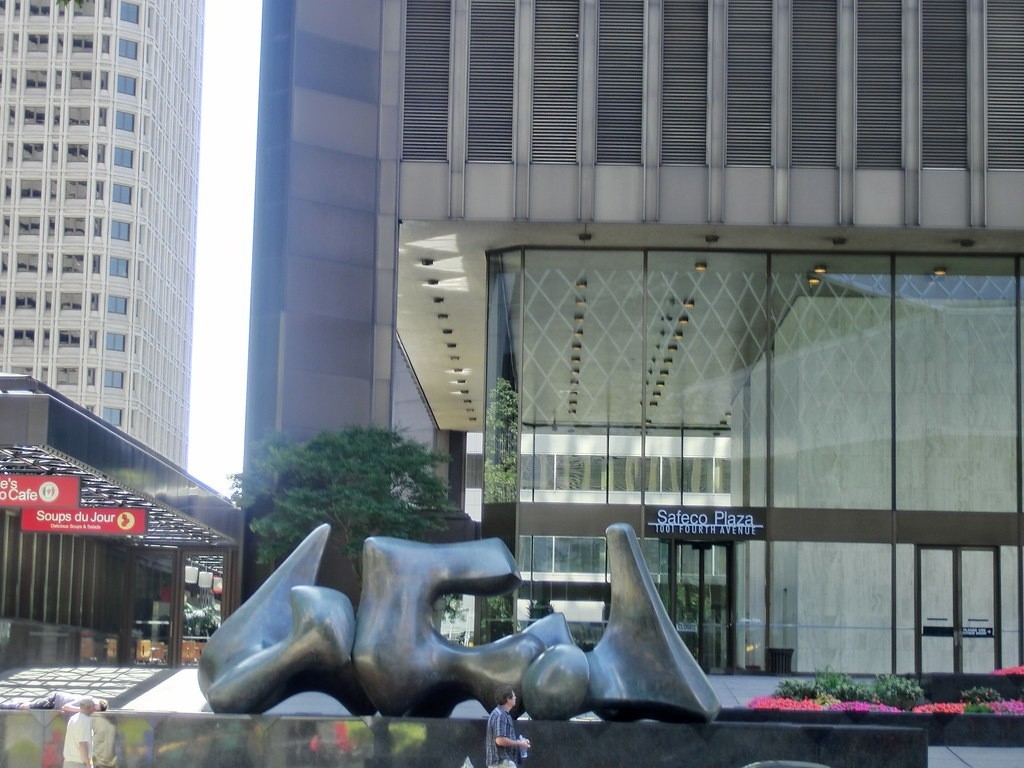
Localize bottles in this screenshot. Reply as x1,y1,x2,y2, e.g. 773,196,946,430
519,735,527,757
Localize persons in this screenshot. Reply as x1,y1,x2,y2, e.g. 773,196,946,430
486,686,530,768
91,716,119,768
62,699,94,768
0,691,108,712
